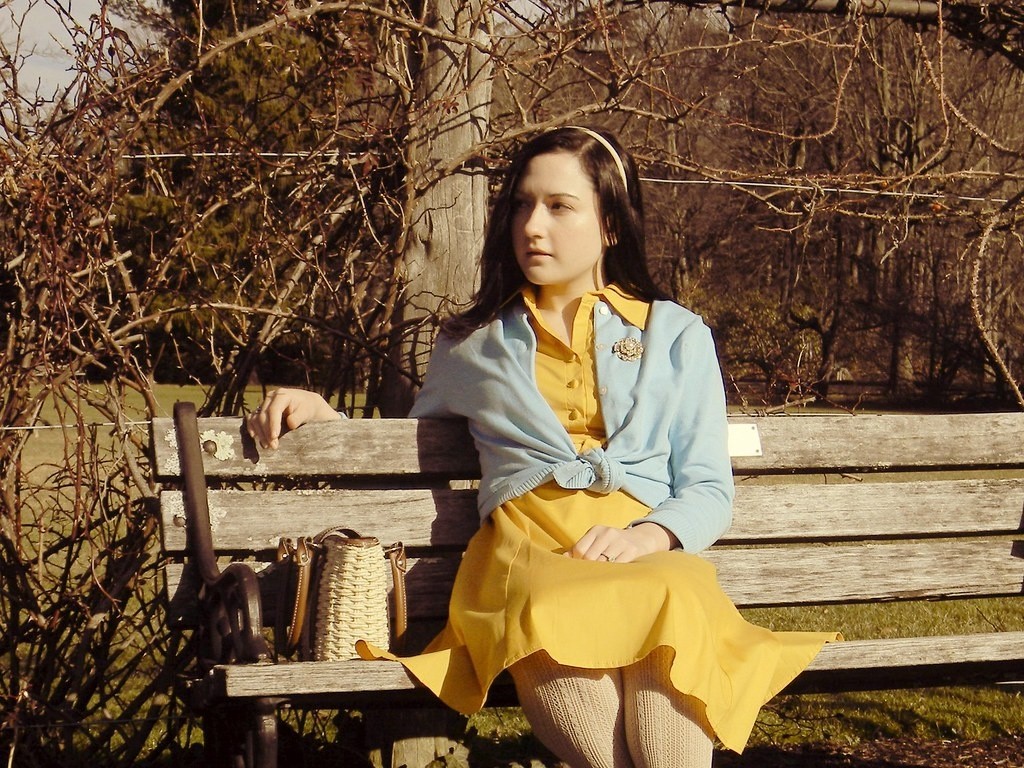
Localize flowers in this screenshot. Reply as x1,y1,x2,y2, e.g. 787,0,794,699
612,336,645,362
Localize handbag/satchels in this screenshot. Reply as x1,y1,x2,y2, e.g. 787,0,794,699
275,524,408,662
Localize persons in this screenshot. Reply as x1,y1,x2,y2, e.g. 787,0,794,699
246,125,847,768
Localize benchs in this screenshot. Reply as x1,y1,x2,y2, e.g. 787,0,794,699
152,412,1024,768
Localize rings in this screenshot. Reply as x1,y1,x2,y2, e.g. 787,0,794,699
601,554,615,562
